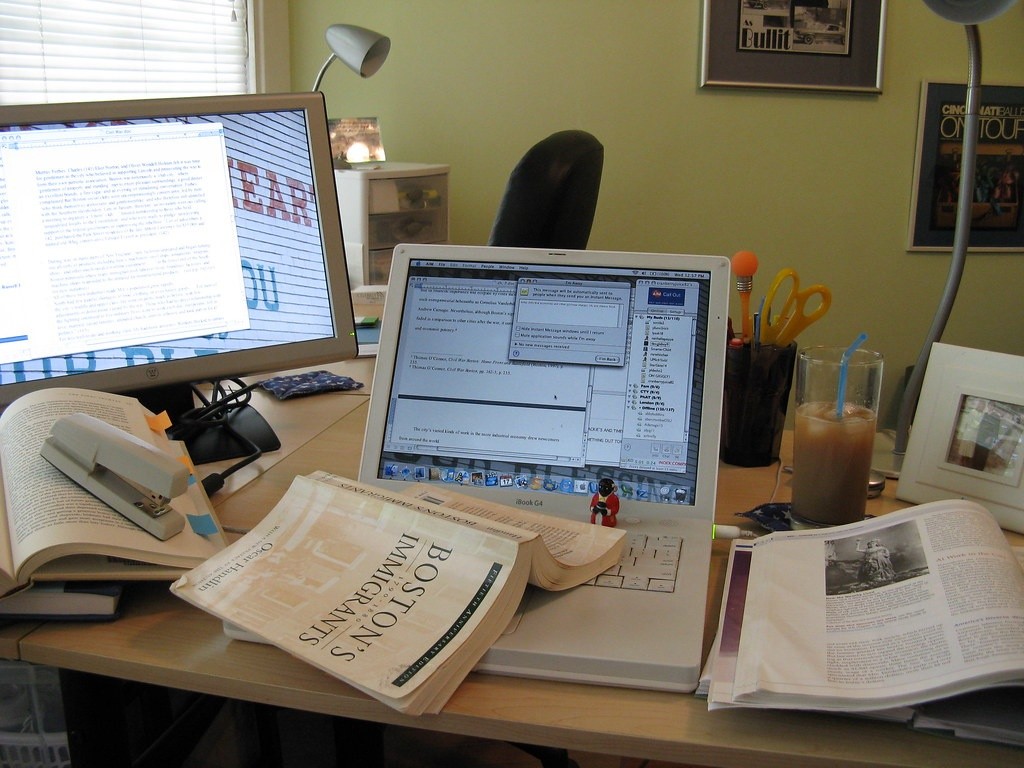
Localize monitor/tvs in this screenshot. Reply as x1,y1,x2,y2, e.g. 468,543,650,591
0,91,359,467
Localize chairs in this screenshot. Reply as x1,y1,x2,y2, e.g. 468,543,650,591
487,129,605,250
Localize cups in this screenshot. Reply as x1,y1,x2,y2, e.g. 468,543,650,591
721,333,798,466
791,345,884,526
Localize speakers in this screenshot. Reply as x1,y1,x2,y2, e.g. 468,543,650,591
487,128,605,251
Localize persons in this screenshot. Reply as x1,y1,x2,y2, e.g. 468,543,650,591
588,478,620,526
961,399,1002,472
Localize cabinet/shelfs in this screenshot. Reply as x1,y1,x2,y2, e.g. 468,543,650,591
333,161,450,288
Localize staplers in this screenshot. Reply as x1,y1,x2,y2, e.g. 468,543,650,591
39,414,189,542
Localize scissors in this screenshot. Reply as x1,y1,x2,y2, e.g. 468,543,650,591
760,268,832,347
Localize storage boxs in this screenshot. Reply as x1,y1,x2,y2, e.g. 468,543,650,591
348,285,389,321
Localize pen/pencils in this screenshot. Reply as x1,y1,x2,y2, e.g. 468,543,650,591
726,318,751,348
732,250,760,345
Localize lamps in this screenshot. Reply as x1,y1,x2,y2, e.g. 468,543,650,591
312,24,391,92
870,0,1023,480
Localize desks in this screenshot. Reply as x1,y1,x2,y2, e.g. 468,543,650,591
0,357,1024,768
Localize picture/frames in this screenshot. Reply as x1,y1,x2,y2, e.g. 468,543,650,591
906,79,1024,253
895,342,1024,535
700,0,889,97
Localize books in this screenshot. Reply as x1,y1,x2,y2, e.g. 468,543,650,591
170,468,627,718
693,490,1023,750
0,388,227,622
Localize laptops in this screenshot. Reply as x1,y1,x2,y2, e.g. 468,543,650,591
224,242,731,694
